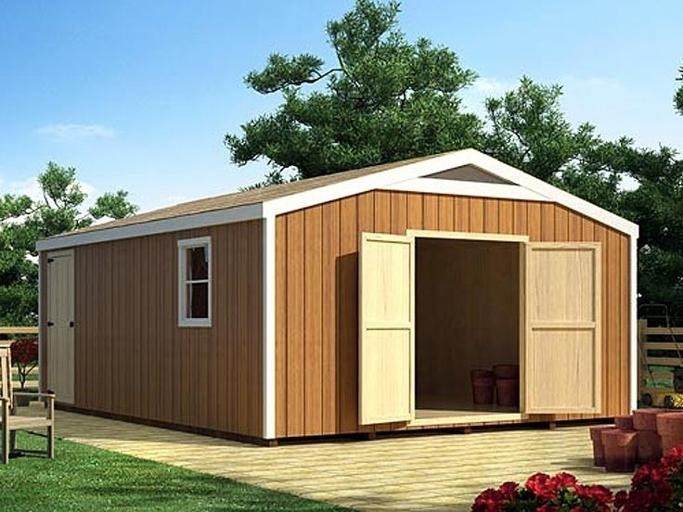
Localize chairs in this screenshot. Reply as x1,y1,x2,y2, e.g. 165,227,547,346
0,347,57,464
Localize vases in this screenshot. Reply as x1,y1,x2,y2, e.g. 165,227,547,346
15,395,31,407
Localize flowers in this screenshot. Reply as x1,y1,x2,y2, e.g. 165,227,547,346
10,339,38,395
